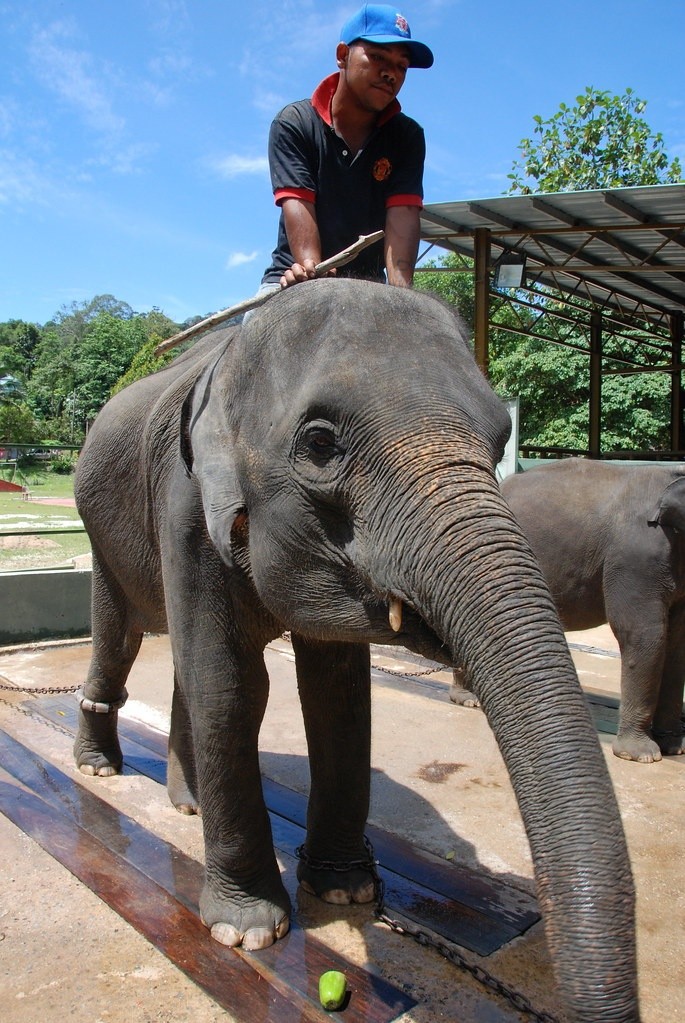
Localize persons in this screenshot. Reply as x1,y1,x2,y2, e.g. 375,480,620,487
239,4,434,329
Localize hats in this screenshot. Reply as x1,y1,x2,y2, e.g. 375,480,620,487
341,3,434,69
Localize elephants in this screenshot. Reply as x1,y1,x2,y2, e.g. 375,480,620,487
70,269,685,1022
